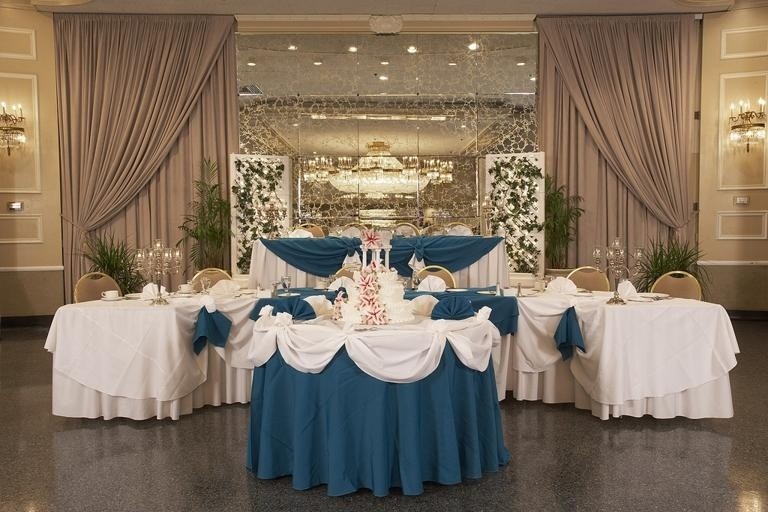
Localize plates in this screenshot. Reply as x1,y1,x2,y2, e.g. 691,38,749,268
101,296,125,300
125,292,144,298
177,289,195,293
277,292,300,298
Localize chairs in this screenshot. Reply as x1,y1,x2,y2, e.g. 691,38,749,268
650,270,702,301
566,266,610,291
412,264,456,289
72,272,123,302
189,268,234,292
296,222,474,237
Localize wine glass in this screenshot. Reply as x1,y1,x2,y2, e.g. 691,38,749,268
201,277,211,294
280,276,293,296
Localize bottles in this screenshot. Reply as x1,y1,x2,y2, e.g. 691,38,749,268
517,283,523,297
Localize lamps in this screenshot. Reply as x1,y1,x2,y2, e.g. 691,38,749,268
0,100,26,157
727,96,768,148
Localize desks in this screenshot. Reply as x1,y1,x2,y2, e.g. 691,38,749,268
252,237,508,287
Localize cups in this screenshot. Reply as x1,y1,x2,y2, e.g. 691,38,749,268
100,289,120,299
179,284,193,291
535,281,546,290
